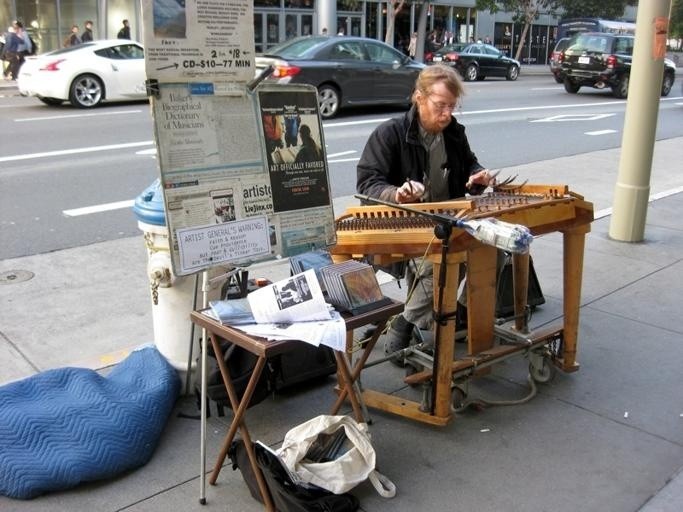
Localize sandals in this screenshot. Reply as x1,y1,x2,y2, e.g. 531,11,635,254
385,323,411,367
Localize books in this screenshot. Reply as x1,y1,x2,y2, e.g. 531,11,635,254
208,295,257,325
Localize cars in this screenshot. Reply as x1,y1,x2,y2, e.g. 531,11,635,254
253,34,430,122
424,42,521,82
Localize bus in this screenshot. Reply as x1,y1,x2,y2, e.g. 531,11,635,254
556,16,635,37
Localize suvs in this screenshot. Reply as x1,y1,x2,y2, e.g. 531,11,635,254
556,29,676,102
548,37,578,85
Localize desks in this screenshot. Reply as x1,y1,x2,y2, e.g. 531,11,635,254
190,295,409,512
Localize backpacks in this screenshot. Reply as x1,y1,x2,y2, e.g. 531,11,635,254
193,268,278,410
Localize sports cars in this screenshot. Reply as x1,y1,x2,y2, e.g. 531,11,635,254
15,39,148,107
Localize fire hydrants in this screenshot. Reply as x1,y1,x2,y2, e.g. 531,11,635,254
132,178,239,395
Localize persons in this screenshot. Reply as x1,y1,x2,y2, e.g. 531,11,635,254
321,28,327,36
336,27,345,37
406,32,417,63
351,63,499,366
0,20,96,82
117,18,131,43
292,126,321,166
427,31,497,52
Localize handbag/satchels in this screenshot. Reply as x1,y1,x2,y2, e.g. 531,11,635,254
227,413,377,512
